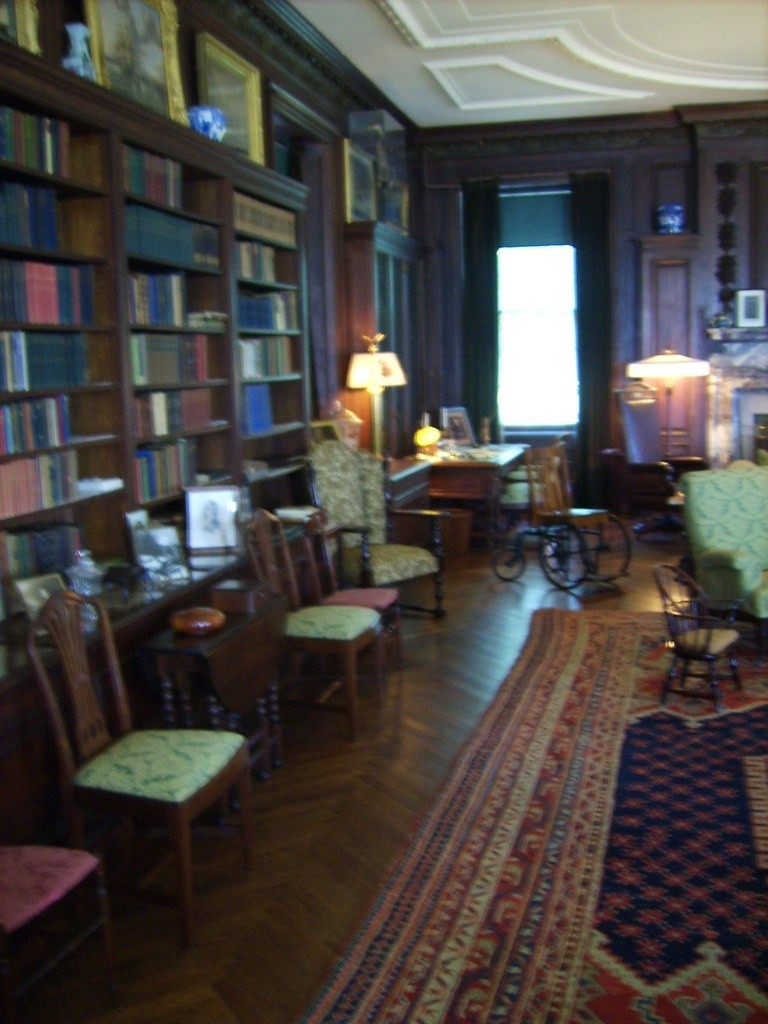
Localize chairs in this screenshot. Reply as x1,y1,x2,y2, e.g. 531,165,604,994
0,509,399,1024
623,397,709,535
677,458,768,667
494,446,631,591
654,563,744,712
305,440,452,617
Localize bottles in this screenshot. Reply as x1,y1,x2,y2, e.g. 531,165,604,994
478,413,491,444
62,22,97,81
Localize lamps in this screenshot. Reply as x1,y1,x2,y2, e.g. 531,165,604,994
627,349,710,455
347,332,406,458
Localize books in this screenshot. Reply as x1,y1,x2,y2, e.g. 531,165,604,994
0,108,299,614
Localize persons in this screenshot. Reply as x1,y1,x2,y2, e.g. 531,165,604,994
196,499,226,547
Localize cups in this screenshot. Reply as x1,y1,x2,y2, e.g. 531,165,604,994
187,105,228,142
655,202,685,234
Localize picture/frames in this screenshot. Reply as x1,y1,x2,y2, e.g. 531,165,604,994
186,485,243,553
737,290,765,328
133,524,189,582
0,0,43,54
85,0,189,126
196,32,264,167
442,407,475,445
345,139,408,238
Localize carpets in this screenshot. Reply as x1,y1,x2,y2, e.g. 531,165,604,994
295,608,768,1024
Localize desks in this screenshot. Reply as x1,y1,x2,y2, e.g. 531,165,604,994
0,509,326,845
406,444,531,566
142,593,285,824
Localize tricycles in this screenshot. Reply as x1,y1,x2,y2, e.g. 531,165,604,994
477,476,632,590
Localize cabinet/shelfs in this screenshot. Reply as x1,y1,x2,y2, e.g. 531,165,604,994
0,38,311,622
344,220,427,454
384,458,433,545
